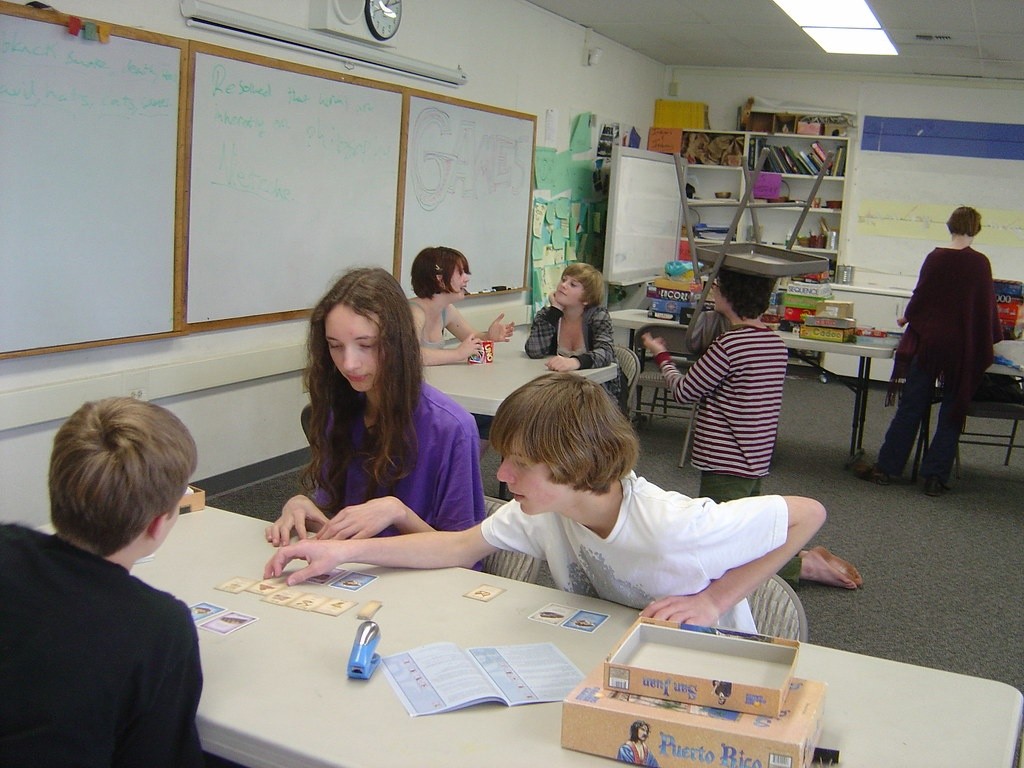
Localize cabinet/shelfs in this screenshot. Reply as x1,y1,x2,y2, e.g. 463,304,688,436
682,129,852,368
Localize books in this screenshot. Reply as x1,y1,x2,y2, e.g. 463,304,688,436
749,138,847,176
379,642,586,718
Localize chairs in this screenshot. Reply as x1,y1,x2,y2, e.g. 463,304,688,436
613,344,641,407
630,322,703,468
747,574,808,643
956,374,1024,480
482,494,540,588
672,147,835,354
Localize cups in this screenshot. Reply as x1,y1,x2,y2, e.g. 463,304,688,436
812,197,821,208
822,231,838,250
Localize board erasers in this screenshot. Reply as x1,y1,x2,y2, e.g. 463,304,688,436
492,286,507,291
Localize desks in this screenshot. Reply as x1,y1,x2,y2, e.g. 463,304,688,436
892,350,1024,483
35,503,1024,768
609,309,894,469
819,282,939,386
421,340,617,498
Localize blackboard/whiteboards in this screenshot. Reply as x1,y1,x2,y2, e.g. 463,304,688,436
0,0,536,361
605,147,688,285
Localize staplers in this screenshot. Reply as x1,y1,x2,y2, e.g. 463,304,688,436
347,621,380,680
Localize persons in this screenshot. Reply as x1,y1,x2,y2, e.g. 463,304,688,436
0,395,211,768
862,207,1003,495
403,247,515,365
642,267,862,592
264,269,489,573
264,370,827,636
525,262,623,394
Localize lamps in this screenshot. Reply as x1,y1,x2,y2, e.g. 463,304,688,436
180,0,468,87
588,47,602,66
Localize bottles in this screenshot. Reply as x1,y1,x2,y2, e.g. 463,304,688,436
785,234,791,246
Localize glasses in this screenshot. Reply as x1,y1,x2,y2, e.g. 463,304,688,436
711,282,720,289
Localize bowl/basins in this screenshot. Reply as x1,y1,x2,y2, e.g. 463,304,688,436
826,200,842,209
714,192,731,198
767,196,789,203
798,236,810,247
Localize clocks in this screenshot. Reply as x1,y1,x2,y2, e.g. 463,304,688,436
365,0,403,42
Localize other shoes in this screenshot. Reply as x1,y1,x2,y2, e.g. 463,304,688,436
855,466,890,485
925,486,944,496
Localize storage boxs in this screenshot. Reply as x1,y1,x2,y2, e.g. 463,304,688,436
821,123,850,137
653,98,708,129
797,121,822,135
179,485,205,514
993,279,1024,341
560,616,829,768
646,241,901,346
648,127,682,154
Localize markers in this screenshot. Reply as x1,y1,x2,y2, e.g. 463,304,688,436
469,289,497,294
508,287,518,290
